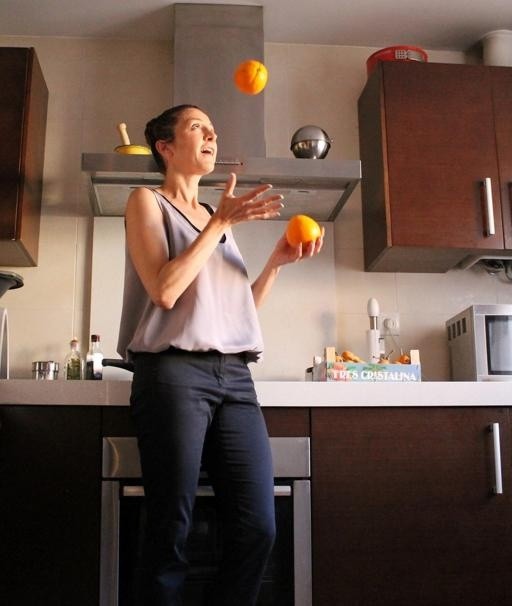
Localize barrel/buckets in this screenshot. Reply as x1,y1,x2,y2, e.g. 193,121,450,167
482,28,511,65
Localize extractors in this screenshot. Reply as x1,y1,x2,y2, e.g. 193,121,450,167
77,3,365,225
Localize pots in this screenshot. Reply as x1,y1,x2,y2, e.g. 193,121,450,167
109,121,153,156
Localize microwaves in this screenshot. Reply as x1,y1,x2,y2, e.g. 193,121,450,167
445,302,512,381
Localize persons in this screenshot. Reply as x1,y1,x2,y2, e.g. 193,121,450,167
117,105,326,605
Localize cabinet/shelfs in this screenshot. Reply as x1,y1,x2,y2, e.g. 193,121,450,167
357,59,511,272
311,408,511,606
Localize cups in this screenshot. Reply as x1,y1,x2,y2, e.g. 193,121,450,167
31,360,59,380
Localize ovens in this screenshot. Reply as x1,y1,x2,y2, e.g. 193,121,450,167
100,432,314,606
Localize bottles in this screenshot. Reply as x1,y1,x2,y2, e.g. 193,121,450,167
63,339,84,380
84,334,104,380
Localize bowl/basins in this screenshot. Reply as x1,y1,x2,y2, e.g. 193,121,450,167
365,44,429,79
